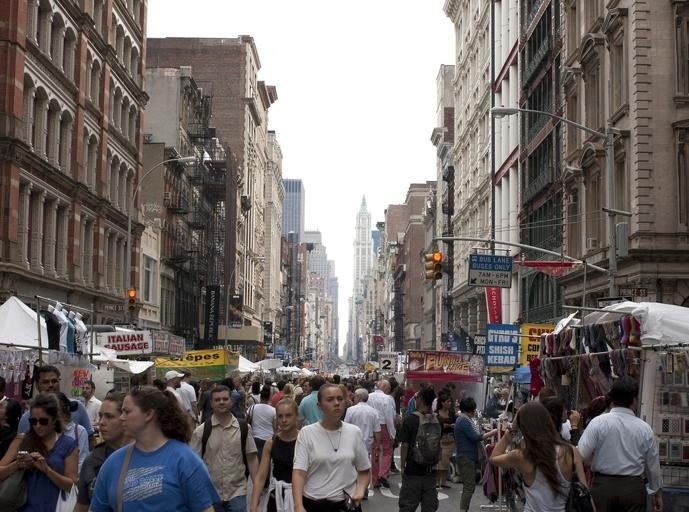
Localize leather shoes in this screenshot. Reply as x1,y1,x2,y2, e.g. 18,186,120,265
377,476,389,488
440,485,450,488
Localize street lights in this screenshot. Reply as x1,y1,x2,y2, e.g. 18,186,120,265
258,306,283,344
225,256,261,344
489,105,620,298
124,154,199,322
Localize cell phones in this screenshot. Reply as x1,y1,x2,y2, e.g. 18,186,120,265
18,451,34,463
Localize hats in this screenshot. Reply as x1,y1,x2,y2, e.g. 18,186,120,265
165,371,185,382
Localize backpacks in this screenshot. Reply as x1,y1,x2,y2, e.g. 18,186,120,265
411,411,442,463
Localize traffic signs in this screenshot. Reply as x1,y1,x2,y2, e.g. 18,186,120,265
596,297,634,309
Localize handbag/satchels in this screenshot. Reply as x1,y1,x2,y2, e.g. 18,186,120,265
565,446,593,512
1,467,28,511
246,405,254,427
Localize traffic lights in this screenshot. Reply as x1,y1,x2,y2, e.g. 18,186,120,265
425,253,434,279
432,251,444,280
127,288,137,312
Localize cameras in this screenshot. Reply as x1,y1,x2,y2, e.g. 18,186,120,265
567,411,573,420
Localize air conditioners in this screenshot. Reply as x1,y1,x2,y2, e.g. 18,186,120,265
587,238,597,252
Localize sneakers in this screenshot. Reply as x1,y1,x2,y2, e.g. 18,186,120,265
391,468,399,473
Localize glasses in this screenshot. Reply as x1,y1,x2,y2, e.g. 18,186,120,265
132,385,141,395
40,378,61,386
29,417,50,426
342,490,356,511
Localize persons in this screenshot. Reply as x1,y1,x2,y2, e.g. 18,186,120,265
575,378,664,512
1,370,608,512
